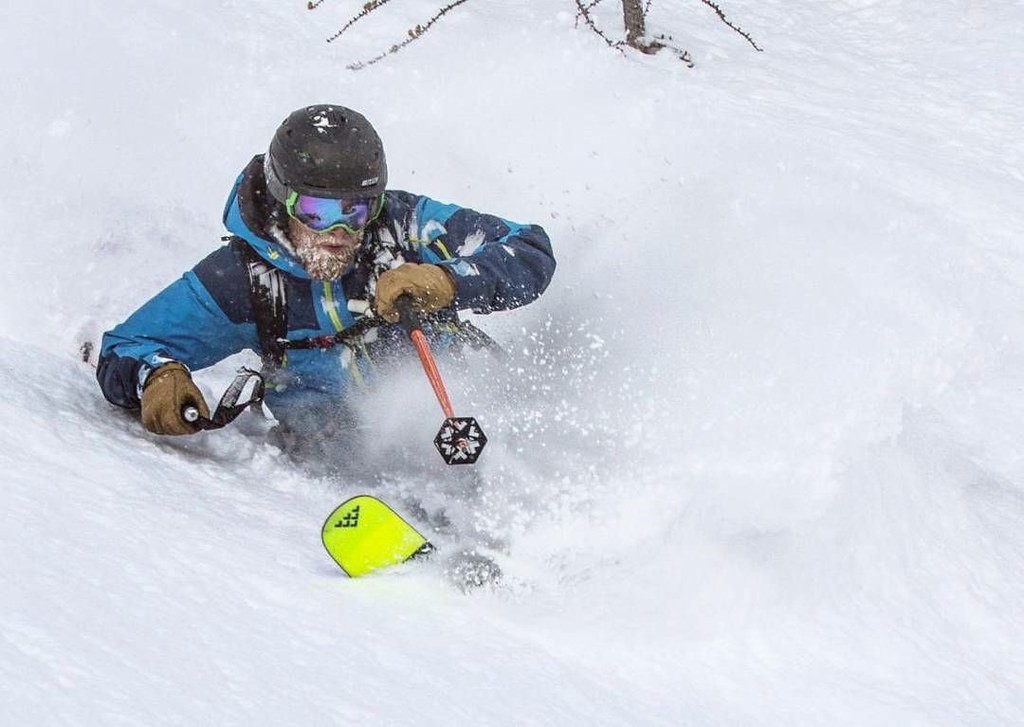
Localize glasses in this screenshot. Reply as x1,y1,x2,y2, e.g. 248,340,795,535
284,191,386,236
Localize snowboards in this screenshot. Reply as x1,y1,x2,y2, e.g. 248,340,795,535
318,493,504,606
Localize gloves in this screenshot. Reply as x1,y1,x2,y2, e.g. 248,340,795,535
140,362,211,436
372,262,457,324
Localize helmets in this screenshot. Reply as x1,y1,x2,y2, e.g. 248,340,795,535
265,104,388,222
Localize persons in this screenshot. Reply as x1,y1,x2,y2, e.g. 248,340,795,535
96,103,559,550
